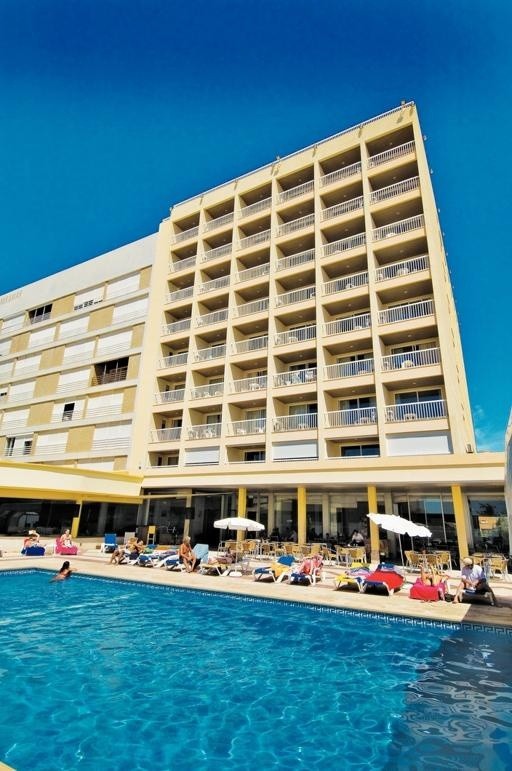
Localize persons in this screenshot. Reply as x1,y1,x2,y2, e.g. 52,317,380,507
110,534,144,565
297,553,324,578
61,529,73,549
25,532,48,549
270,528,283,541
452,558,499,607
49,560,79,584
180,536,197,574
208,550,244,565
348,530,366,547
329,537,337,544
324,533,329,543
287,529,297,543
417,564,450,587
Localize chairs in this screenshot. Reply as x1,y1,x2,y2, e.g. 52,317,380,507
102,532,508,605
22,534,78,558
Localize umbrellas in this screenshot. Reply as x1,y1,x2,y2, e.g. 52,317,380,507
365,513,433,582
213,517,265,574
